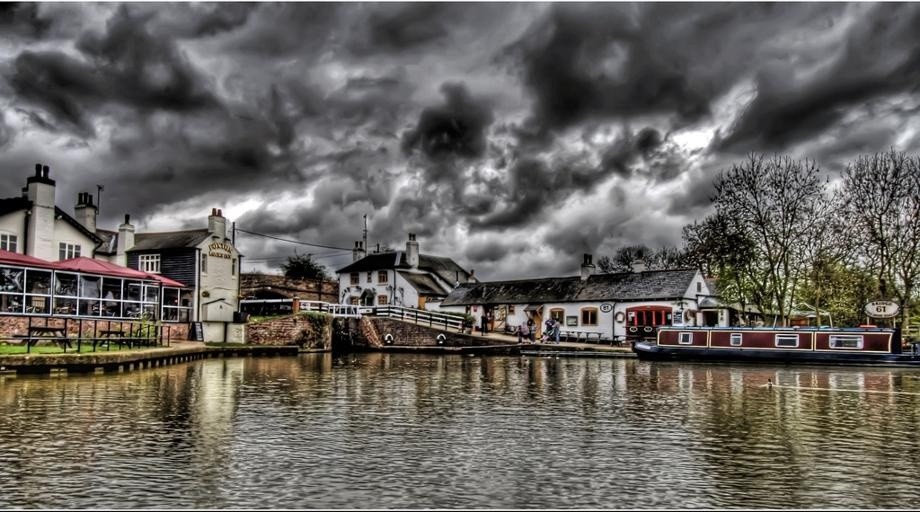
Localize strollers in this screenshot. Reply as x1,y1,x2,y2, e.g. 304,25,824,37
541,327,556,344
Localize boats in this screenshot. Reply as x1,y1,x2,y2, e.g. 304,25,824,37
629,325,920,368
